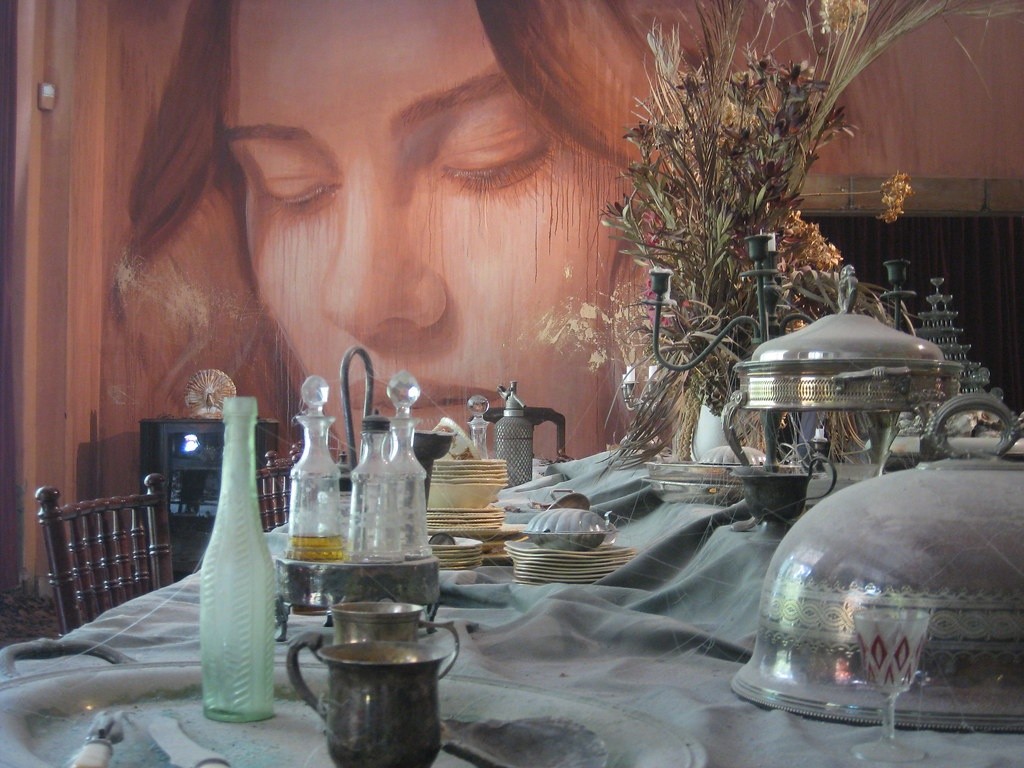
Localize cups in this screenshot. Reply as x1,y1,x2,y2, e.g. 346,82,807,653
738,455,839,541
329,600,461,682
430,417,481,462
285,630,453,768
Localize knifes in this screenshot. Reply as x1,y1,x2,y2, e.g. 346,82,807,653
144,715,232,768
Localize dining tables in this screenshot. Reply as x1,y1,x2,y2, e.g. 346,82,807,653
0,433,1023,768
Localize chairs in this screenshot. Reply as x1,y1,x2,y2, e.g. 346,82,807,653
253,443,307,534
33,470,176,637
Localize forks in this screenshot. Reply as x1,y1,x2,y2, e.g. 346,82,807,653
66,708,137,767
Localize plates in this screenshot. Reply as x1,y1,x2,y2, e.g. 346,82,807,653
643,459,769,484
425,454,645,592
640,480,747,508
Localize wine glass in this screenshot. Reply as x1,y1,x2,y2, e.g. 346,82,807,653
914,276,1002,398
850,608,930,762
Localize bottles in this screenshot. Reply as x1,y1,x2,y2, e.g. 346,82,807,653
493,380,534,489
343,410,402,564
283,374,346,562
464,394,491,461
381,368,434,561
197,396,278,724
